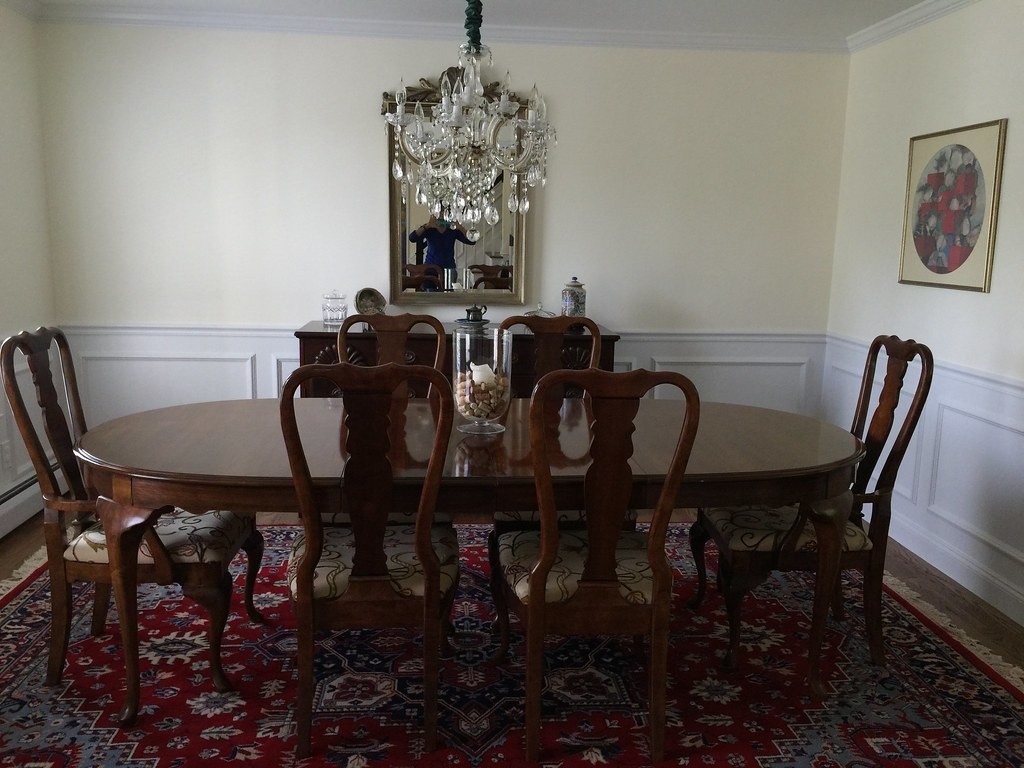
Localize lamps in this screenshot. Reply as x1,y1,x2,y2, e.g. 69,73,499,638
380,0,560,244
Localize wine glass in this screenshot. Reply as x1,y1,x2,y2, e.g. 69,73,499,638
454,329,513,435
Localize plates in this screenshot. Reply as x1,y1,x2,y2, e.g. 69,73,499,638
454,319,489,329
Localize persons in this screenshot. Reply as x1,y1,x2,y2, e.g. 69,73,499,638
409,199,477,293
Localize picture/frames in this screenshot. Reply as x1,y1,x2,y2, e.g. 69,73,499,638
895,117,1011,295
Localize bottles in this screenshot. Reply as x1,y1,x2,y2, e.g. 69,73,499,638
558,389,590,460
525,303,555,335
562,276,586,319
321,295,348,325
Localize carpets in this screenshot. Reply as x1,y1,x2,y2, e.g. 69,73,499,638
0,517,1024,768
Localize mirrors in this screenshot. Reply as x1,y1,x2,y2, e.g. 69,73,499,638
376,68,538,307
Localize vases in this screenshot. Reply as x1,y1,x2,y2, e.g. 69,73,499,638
452,326,513,435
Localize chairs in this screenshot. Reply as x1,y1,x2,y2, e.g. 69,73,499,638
405,262,444,289
328,313,450,399
486,361,703,763
473,275,514,289
672,334,936,692
467,263,513,284
488,313,610,394
285,360,463,760
0,325,262,694
402,274,444,291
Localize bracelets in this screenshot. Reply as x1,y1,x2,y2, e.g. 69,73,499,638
419,223,427,231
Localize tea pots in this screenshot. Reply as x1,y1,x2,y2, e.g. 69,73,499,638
465,304,487,322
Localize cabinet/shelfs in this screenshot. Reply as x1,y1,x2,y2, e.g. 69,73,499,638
291,319,623,400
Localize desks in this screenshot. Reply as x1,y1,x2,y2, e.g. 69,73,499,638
74,397,870,730
404,287,513,293
